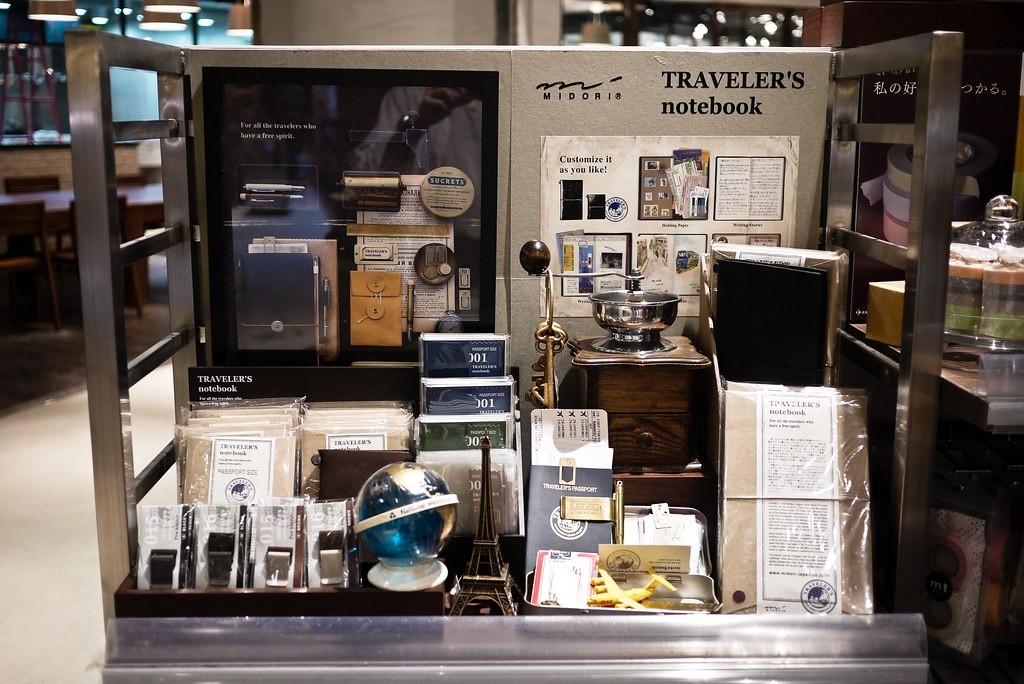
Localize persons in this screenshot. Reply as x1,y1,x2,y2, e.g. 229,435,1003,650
350,85,482,238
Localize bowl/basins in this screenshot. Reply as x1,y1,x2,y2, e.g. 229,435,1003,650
590,291,682,353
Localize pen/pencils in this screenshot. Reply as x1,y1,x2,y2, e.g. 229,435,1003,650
313,255,322,366
321,277,329,343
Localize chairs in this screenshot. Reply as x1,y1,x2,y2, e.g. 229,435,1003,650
0,172,170,336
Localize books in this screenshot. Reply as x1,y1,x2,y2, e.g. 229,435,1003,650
708,243,874,615
175,333,518,536
846,49,1022,322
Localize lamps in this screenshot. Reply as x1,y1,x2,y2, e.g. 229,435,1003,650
28,0,255,38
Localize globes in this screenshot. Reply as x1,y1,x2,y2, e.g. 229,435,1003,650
354,462,459,593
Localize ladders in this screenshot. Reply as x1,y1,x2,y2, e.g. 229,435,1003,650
0,0,67,144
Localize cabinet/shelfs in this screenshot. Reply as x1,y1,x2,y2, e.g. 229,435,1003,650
839,322,1023,684
566,330,716,555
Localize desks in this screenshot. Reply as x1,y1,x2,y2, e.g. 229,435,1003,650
0,186,164,305
